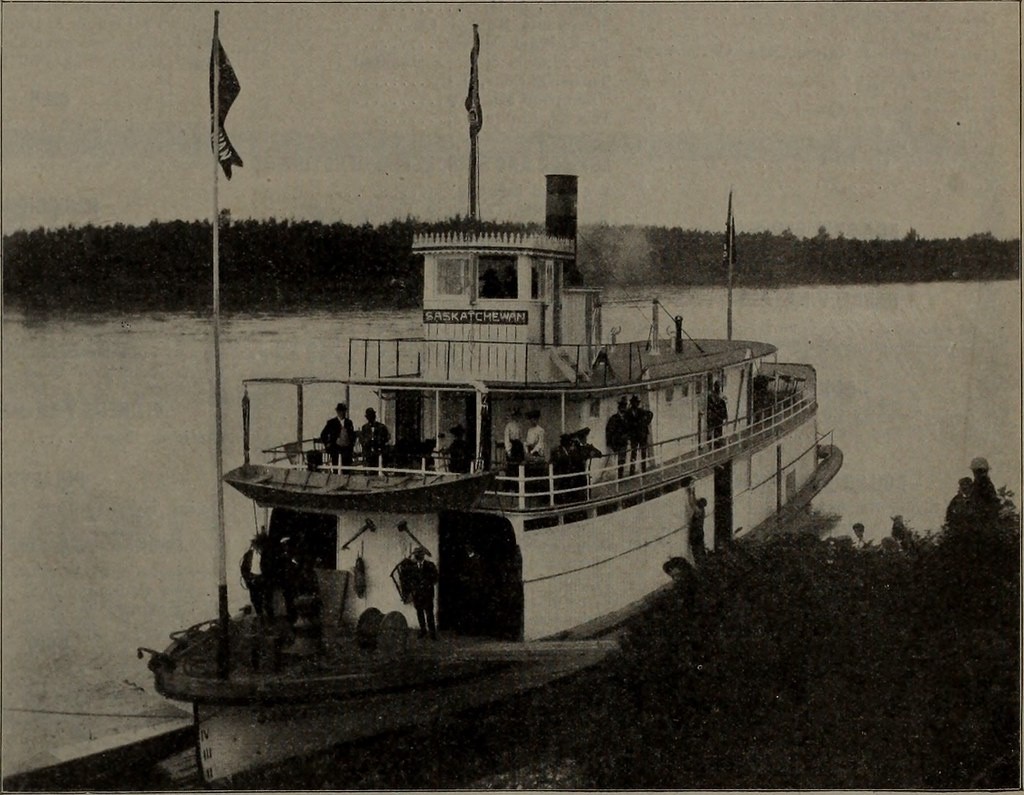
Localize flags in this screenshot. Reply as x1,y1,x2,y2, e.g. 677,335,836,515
209,35,244,183
465,28,484,139
721,197,737,269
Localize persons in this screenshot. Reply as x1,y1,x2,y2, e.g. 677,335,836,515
444,424,472,472
504,409,544,498
705,389,729,449
481,262,538,298
687,483,707,557
947,457,1000,525
410,548,438,640
321,403,356,474
606,397,654,481
359,408,391,466
241,534,328,665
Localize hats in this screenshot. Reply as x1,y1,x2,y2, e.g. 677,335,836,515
364,407,377,415
410,546,427,555
335,403,349,410
616,400,628,406
968,457,991,468
630,396,642,402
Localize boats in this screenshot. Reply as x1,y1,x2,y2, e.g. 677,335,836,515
135,190,846,793
